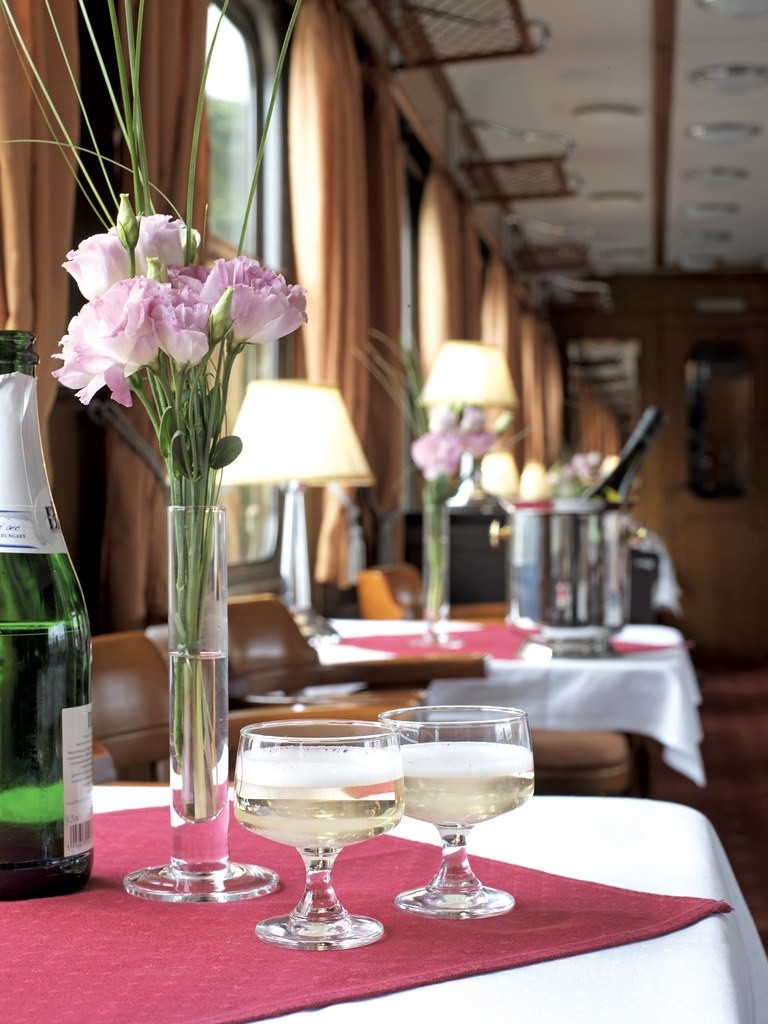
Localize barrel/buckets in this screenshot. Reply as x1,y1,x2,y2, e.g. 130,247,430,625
507,497,631,659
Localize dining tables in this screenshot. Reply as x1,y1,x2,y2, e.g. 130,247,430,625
303,615,707,787
0,785,768,1024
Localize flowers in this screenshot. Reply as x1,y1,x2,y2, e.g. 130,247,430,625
0,0,310,505
352,322,530,498
550,447,613,496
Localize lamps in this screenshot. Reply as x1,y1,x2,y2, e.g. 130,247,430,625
413,339,521,513
587,191,640,212
682,165,749,192
693,0,768,19
683,202,739,224
568,103,642,122
682,121,762,144
605,247,646,265
673,253,726,273
683,63,768,96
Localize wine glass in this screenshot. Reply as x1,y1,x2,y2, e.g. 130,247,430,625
234,716,406,951
379,703,536,921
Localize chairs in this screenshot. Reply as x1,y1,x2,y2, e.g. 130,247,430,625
356,562,420,620
92,626,170,783
221,594,488,708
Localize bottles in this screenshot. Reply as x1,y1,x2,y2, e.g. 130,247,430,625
0,329,94,901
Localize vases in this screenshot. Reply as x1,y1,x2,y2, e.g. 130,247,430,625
122,505,279,901
421,505,451,644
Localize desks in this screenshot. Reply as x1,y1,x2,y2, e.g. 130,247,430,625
401,505,506,605
